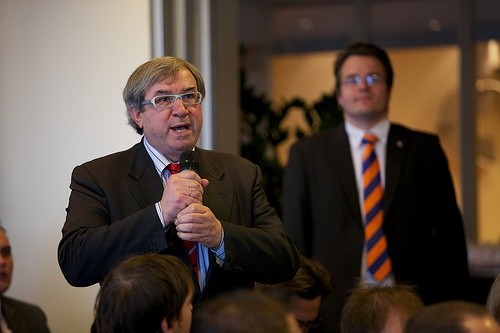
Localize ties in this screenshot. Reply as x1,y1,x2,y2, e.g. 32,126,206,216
165,162,199,284
359,132,392,283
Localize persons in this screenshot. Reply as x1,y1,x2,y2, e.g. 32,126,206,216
282,38,469,307
57,56,300,298
90,253,500,333
0,225,52,333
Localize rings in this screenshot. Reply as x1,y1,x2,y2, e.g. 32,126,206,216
189,188,192,196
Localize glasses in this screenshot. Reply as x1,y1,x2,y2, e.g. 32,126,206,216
336,73,389,87
140,92,203,109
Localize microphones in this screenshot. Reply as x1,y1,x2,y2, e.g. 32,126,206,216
180,151,200,175
